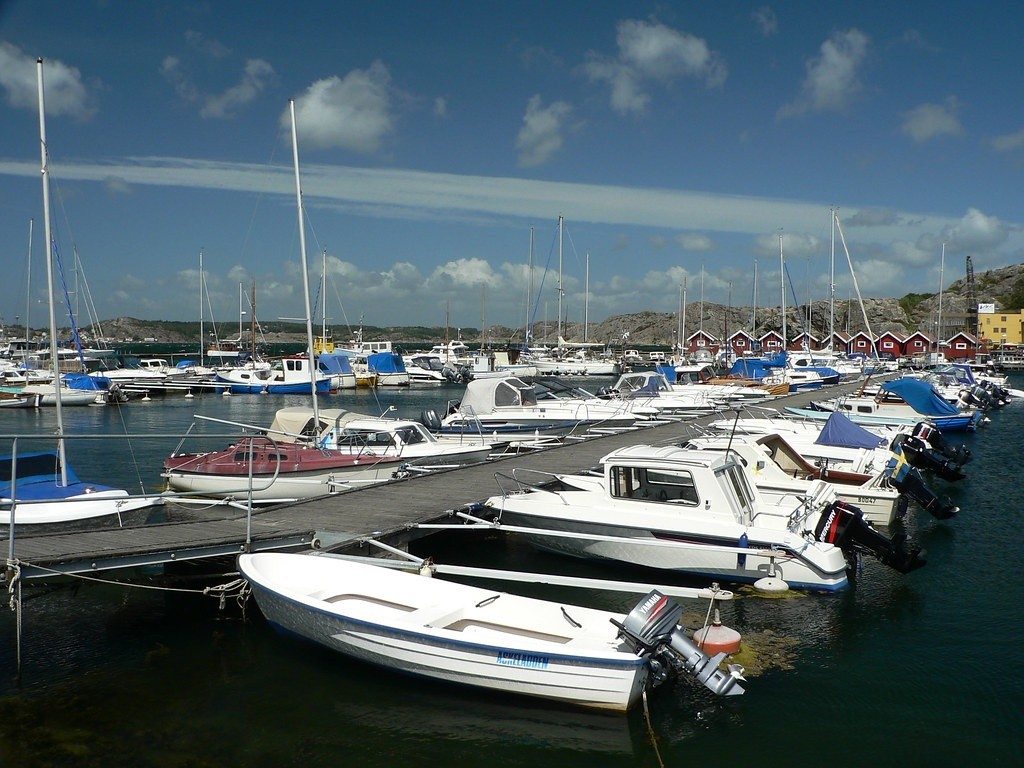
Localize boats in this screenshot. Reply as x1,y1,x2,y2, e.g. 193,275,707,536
692,364,1013,547
435,372,769,433
274,407,508,466
484,442,852,596
236,549,744,713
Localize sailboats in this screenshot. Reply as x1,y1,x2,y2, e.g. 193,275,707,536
152,100,404,499
0,203,946,396
0,56,163,528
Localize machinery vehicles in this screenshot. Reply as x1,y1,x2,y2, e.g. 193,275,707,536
965,256,978,335
